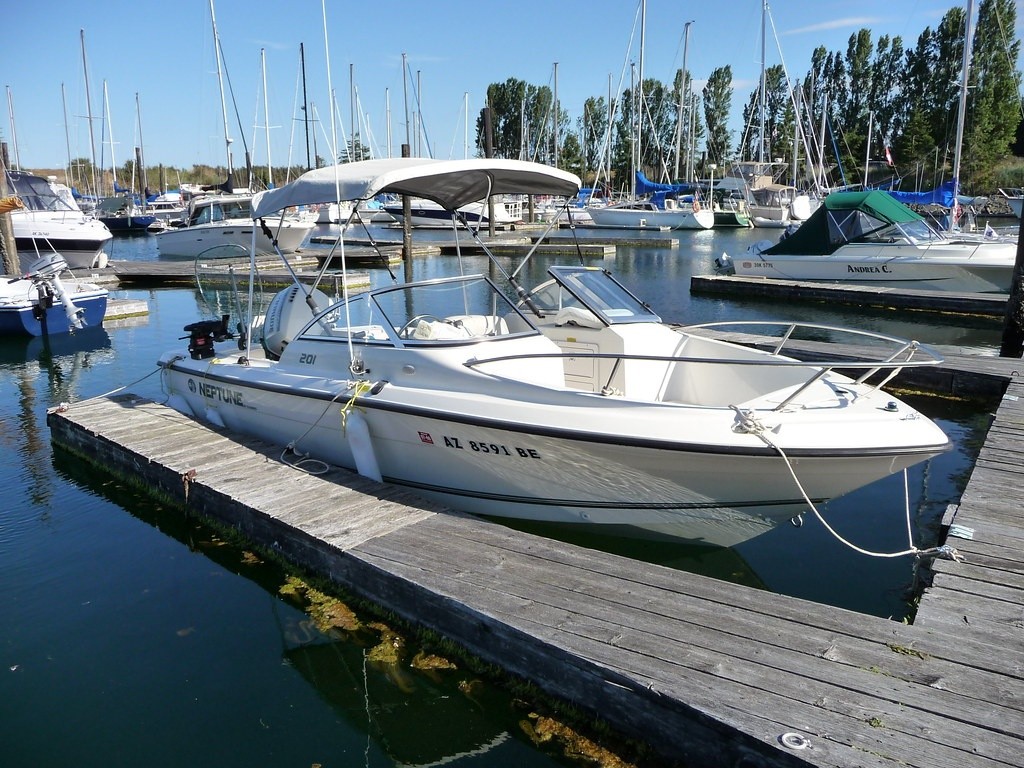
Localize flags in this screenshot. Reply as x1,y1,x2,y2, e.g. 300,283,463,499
886,149,892,165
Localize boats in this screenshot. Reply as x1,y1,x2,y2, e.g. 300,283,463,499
0,1,812,340
157,150,958,549
725,190,1023,296
